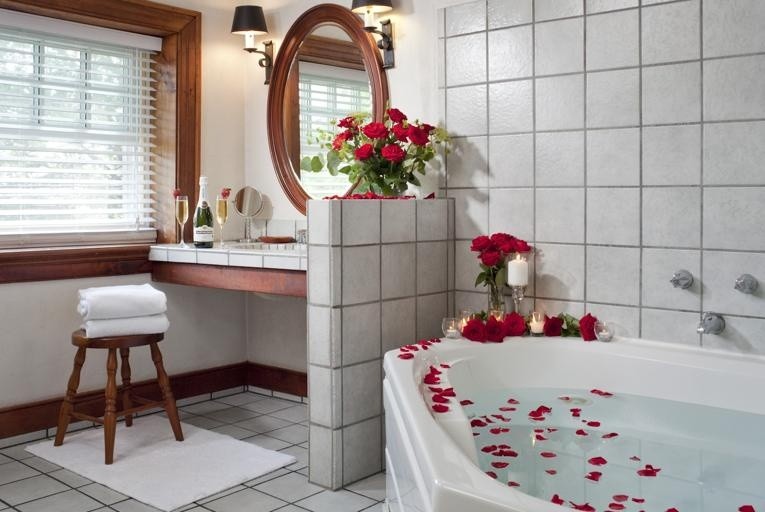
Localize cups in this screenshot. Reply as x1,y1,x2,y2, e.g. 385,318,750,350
457,308,477,335
530,312,547,337
595,322,615,341
442,318,461,337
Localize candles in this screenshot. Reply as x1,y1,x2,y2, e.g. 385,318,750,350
460,315,474,334
508,252,529,287
446,321,457,337
530,315,546,337
598,321,610,342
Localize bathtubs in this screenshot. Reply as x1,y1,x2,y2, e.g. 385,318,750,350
382,333,765,512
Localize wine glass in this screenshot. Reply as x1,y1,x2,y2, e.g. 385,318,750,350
217,196,227,248
176,196,188,246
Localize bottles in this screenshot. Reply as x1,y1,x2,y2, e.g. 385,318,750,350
193,175,214,249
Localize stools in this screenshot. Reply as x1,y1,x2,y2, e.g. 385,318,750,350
54,329,184,465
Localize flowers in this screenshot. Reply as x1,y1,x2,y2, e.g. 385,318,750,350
220,187,231,199
326,108,453,197
461,316,599,344
173,189,182,199
470,233,531,310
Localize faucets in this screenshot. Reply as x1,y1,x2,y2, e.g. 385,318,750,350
696,313,722,335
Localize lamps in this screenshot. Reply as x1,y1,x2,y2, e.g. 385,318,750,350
231,5,269,54
351,0,393,33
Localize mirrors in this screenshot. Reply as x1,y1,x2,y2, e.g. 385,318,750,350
231,185,263,243
267,3,388,216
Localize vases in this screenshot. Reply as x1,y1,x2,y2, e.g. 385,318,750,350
488,283,506,321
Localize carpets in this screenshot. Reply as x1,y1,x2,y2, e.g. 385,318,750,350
25,414,297,512
